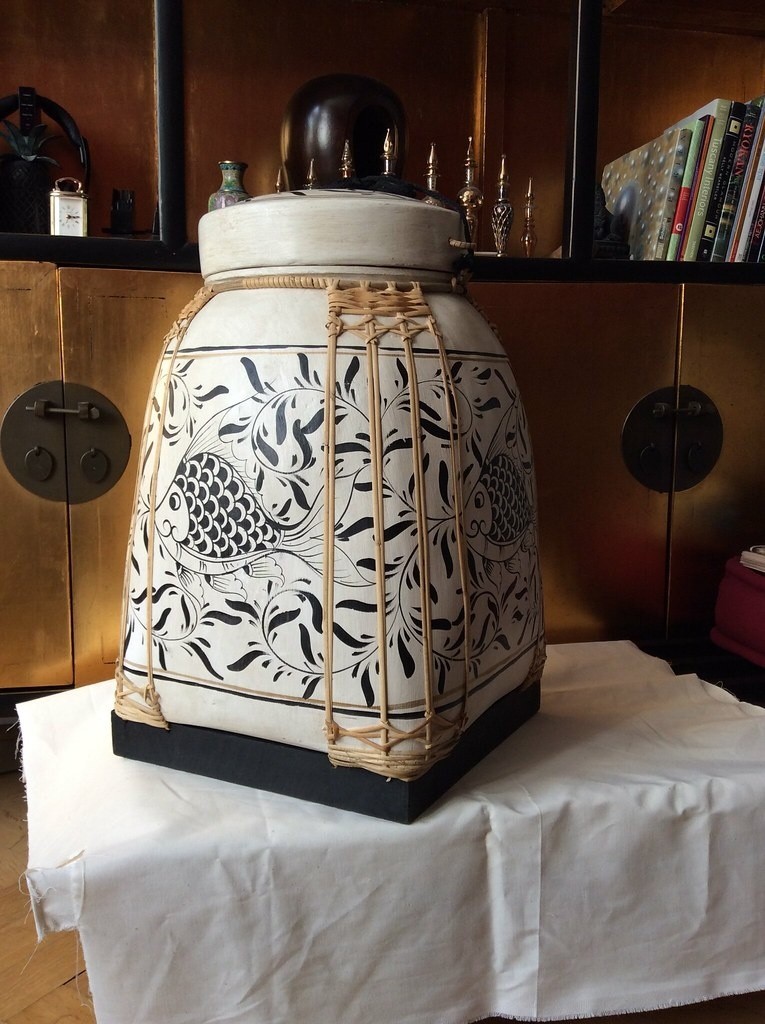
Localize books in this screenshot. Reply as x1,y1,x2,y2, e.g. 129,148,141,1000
601,97,764,262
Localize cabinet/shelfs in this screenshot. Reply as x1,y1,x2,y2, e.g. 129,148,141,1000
0,0,765,696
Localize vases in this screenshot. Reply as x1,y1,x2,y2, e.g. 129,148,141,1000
208,160,251,213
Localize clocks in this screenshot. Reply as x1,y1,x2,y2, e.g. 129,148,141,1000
49,177,88,238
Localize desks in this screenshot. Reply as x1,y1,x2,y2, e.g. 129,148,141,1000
6,639,765,1024
